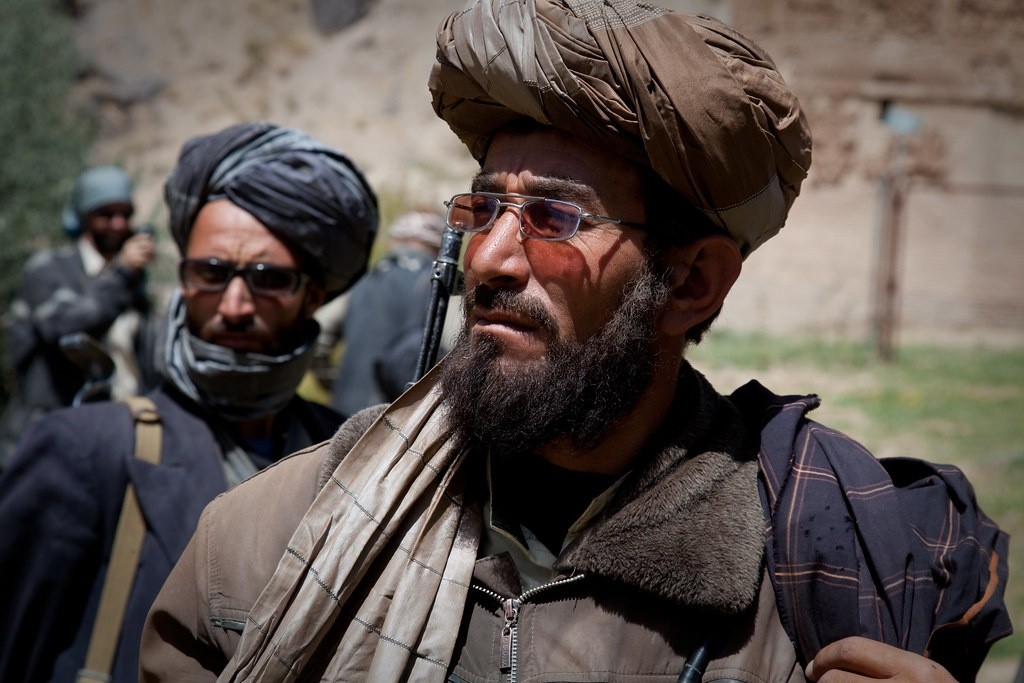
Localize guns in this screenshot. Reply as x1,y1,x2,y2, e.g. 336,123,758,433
402,222,465,391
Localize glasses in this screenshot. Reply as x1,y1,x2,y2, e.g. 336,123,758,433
443,192,656,244
178,257,308,296
93,207,132,219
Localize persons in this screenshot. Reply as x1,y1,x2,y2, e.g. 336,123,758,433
137,1,1014,683
0,121,382,683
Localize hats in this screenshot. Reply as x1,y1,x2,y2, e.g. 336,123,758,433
162,120,380,303
387,211,443,248
427,0,813,262
